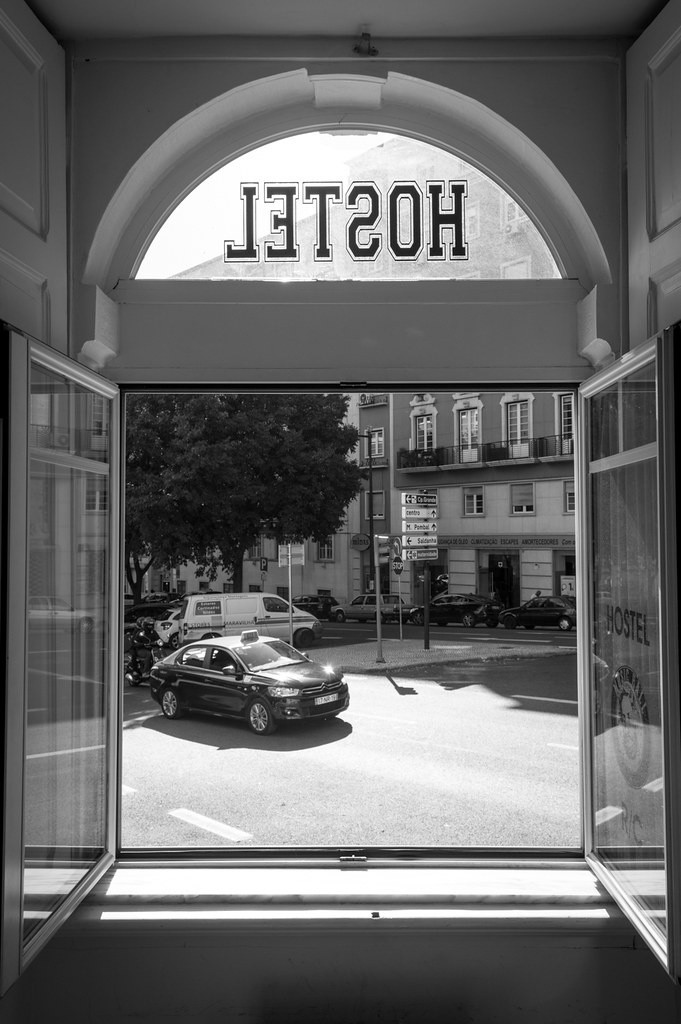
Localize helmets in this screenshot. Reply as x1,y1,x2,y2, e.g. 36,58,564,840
136,617,145,628
142,617,155,630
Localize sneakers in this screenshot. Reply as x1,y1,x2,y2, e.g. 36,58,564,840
142,672,150,677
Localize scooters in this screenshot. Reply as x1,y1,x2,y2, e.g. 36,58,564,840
124,631,167,687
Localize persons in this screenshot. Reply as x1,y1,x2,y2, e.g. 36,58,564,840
531,591,541,600
130,589,164,678
493,588,502,602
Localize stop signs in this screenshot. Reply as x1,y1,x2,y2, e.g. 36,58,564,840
393,556,403,575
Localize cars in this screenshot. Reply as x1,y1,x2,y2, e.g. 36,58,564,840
408,593,506,628
292,594,339,619
498,596,577,631
330,594,406,624
124,604,175,623
124,593,134,606
153,607,182,649
149,630,350,736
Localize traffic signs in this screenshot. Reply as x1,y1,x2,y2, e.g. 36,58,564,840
402,521,438,533
402,535,437,547
401,492,438,506
401,507,437,519
402,548,439,561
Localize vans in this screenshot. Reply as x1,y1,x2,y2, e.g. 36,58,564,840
178,592,323,648
